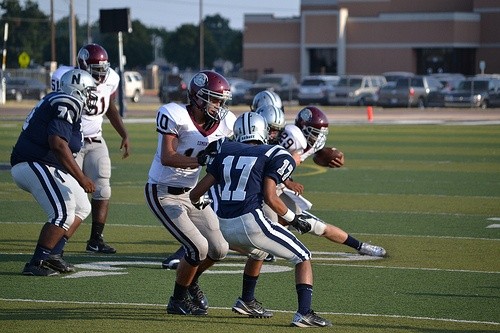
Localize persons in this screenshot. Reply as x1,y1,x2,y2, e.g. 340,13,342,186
11,44,131,274
145,71,388,328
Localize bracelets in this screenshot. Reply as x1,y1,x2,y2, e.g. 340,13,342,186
281,208,295,222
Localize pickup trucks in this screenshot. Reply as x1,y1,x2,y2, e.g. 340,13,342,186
377,75,443,108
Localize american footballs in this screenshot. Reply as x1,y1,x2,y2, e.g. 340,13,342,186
312,147,341,167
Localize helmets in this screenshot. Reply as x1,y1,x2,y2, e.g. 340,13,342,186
189,70,232,120
255,105,286,143
251,90,284,118
295,106,329,147
78,43,110,84
278,124,307,152
60,69,98,113
233,112,270,145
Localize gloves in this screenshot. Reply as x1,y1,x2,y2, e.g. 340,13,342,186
281,208,311,234
190,196,213,209
198,139,222,166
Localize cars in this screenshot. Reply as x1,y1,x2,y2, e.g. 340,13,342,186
383,72,411,85
433,74,466,86
443,75,500,108
224,78,253,106
328,76,387,106
245,75,300,106
124,72,144,102
159,74,194,103
6,78,47,102
298,76,341,106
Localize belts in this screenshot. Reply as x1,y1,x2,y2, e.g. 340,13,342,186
167,185,192,195
84,136,102,143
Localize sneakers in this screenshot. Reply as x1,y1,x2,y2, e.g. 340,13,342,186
188,284,209,310
232,297,274,318
359,242,387,256
291,309,330,327
86,239,116,253
167,296,201,315
23,263,58,276
162,257,181,269
46,255,75,273
